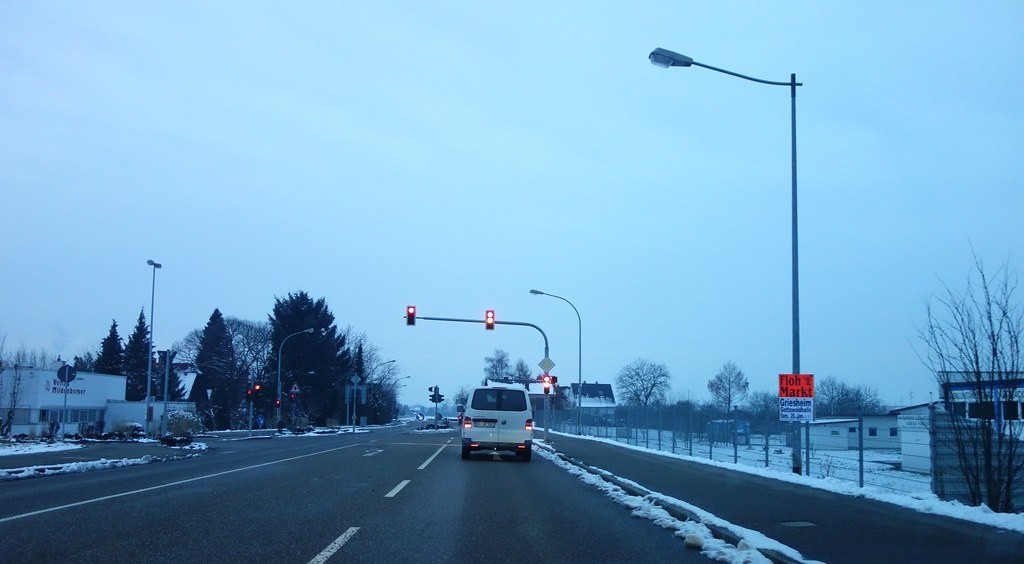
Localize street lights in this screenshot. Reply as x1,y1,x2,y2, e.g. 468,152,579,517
530,288,582,437
392,384,408,418
346,360,397,425
144,259,162,439
648,46,804,474
277,328,314,432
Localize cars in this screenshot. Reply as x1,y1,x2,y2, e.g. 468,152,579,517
456,413,462,425
424,417,448,430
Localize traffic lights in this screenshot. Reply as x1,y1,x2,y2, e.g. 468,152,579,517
543,373,550,394
485,310,495,329
552,375,558,384
254,385,262,390
290,394,296,399
406,306,416,326
247,390,252,401
276,401,280,407
429,395,436,403
437,393,445,403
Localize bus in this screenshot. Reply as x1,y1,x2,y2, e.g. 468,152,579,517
706,419,751,445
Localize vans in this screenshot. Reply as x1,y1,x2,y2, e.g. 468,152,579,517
456,386,536,461
